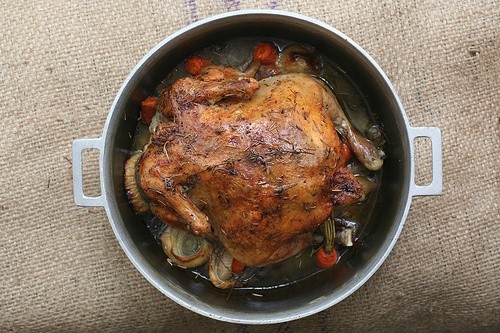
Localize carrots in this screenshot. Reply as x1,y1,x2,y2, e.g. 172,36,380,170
313,218,339,269
140,42,280,125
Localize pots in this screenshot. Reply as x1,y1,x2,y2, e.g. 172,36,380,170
68,8,442,329
